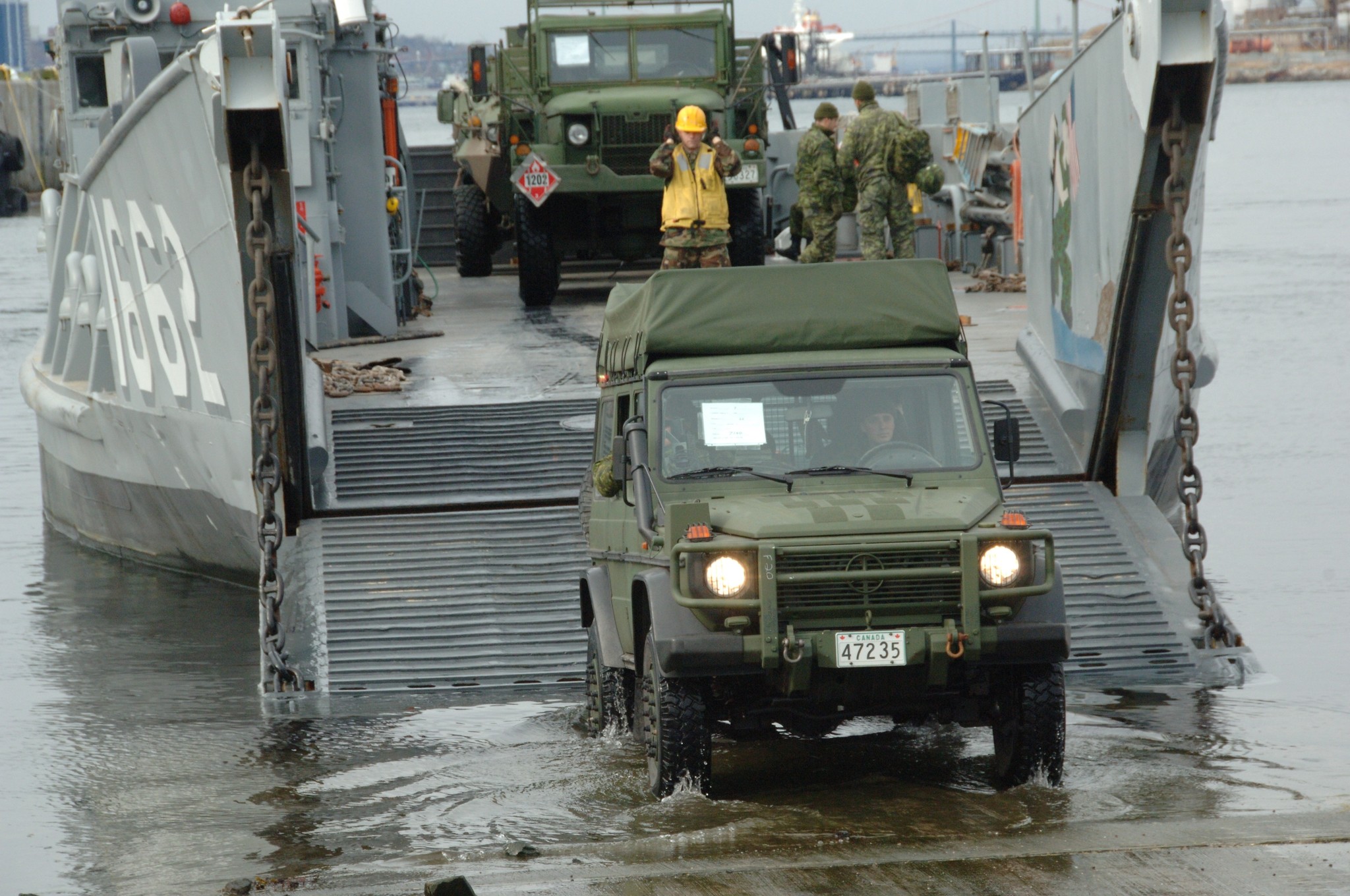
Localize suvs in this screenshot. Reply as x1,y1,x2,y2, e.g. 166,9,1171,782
581,257,1070,802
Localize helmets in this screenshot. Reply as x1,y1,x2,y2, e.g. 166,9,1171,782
674,105,707,133
914,163,944,194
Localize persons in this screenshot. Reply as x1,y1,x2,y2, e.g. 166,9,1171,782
798,101,846,263
843,395,906,470
835,80,913,261
649,106,742,270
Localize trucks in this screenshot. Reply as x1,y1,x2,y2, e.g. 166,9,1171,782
438,0,774,315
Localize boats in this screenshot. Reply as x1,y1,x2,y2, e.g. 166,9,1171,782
15,1,1264,731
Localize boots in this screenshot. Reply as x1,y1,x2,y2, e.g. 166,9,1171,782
775,235,801,262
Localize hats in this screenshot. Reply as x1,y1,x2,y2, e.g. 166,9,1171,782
814,101,839,120
852,80,875,101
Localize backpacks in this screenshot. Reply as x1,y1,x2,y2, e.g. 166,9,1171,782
883,110,935,183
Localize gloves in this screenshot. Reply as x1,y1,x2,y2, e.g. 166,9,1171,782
705,120,721,142
663,124,677,144
831,203,843,221
